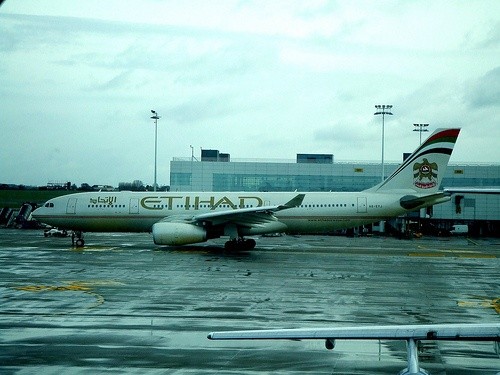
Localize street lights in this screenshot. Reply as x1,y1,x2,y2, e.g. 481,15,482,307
412,123,429,146
150,109,162,192
373,104,394,233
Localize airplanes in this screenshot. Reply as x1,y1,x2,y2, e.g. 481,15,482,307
29,127,463,254
